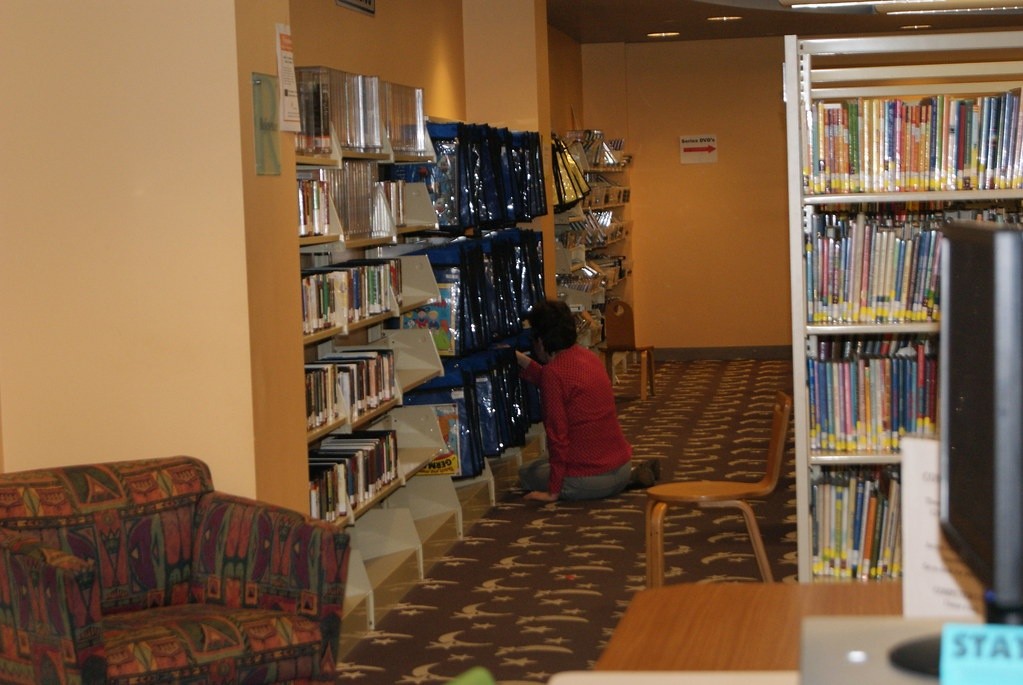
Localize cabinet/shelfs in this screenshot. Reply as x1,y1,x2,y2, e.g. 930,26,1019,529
781,32,1023,585
288,63,636,658
337,0,376,15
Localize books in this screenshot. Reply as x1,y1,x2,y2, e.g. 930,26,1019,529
308,430,399,525
300,258,405,335
802,205,1023,325
296,160,405,237
304,346,396,431
810,463,902,583
799,88,1023,196
291,65,426,157
805,333,940,451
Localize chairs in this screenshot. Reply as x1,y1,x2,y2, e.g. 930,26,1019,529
645,391,793,588
598,298,655,403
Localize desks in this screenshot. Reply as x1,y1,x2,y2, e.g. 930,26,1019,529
594,578,902,672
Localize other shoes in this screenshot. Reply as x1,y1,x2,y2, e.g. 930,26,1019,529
632,459,661,484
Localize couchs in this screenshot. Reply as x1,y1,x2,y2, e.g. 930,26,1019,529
0,456,351,685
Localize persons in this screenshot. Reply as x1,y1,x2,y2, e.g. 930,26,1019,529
497,300,662,504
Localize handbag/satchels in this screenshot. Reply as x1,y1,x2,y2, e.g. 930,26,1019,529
551,134,591,214
